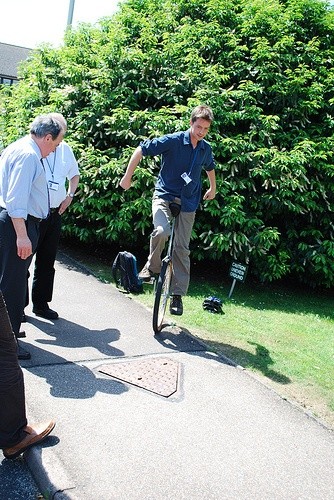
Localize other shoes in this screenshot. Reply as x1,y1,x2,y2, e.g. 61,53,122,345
17,346,31,359
169,295,183,316
20,313,26,322
32,306,58,319
18,329,25,337
138,262,156,281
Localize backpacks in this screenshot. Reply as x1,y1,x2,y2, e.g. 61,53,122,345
112,251,143,293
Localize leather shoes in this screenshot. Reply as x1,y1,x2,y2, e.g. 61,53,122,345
2,420,56,459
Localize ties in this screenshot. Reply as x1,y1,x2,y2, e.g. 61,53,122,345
40,158,50,220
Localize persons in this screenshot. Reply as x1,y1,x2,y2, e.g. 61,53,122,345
119,105,216,333
0,290,56,460
21,113,80,321
0,115,64,359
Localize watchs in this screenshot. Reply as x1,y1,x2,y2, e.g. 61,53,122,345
67,192,75,198
